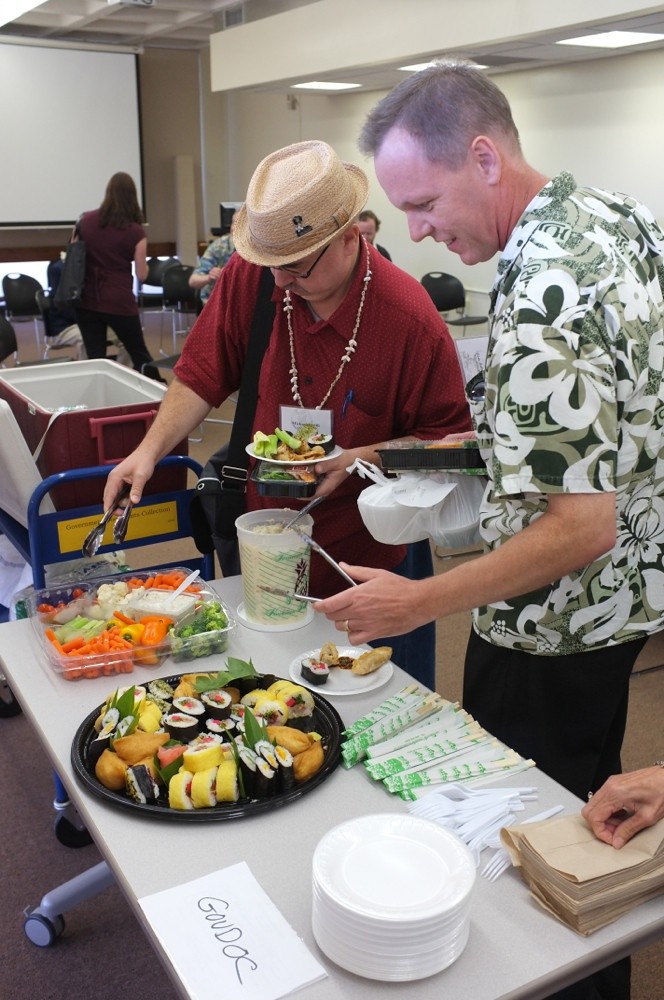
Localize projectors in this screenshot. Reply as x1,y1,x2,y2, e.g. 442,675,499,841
107,0,154,8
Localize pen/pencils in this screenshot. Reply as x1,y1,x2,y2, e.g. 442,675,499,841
342,390,353,416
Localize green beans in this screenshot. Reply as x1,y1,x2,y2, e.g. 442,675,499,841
258,471,296,480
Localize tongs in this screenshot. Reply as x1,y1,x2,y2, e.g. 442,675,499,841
81,487,132,558
257,521,357,603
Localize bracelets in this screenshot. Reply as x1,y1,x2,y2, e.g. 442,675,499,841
652,760,664,767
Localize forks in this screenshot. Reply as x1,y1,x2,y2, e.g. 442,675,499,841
408,781,564,883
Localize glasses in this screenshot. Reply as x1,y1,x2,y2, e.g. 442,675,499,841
273,243,332,279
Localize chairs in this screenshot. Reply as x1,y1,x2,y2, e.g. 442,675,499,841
0,245,488,442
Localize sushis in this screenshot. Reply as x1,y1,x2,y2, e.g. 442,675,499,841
301,643,392,685
308,434,335,452
90,678,328,810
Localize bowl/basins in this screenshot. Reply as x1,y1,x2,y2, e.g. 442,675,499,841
363,494,428,544
440,515,481,549
249,460,319,498
373,439,486,469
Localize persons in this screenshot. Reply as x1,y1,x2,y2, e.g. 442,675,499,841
188,232,236,309
55,173,168,383
356,210,393,262
104,140,479,695
311,58,664,1000
581,760,664,850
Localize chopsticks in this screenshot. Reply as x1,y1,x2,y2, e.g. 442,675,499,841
342,685,536,801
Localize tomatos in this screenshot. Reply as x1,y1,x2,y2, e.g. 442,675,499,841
38,588,83,612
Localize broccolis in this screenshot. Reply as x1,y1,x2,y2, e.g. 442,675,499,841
167,601,228,657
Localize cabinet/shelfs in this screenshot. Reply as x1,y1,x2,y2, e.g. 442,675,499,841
0,455,217,716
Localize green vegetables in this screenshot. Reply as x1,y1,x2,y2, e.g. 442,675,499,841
253,427,301,459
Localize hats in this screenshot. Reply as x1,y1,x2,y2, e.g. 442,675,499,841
232,139,372,267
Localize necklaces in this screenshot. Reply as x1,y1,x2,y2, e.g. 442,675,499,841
283,233,371,409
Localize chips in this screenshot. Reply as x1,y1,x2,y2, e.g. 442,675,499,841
273,441,325,461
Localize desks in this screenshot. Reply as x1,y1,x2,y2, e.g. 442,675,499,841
0,578,664,1000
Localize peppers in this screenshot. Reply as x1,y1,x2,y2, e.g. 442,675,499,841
106,611,174,664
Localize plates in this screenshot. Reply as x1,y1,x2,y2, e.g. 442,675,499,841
70,670,347,823
288,645,394,696
310,814,478,982
245,442,343,467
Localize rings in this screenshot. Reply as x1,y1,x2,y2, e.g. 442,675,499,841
342,619,351,633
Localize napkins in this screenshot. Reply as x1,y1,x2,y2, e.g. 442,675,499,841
499,816,664,937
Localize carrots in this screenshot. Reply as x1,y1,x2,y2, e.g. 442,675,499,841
44,628,134,678
127,571,201,593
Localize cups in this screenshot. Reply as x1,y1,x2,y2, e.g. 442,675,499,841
235,508,314,624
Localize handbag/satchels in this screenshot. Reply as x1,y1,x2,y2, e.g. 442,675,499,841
51,215,87,323
195,442,242,579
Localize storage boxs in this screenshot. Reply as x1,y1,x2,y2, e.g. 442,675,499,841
0,359,189,531
22,562,236,682
